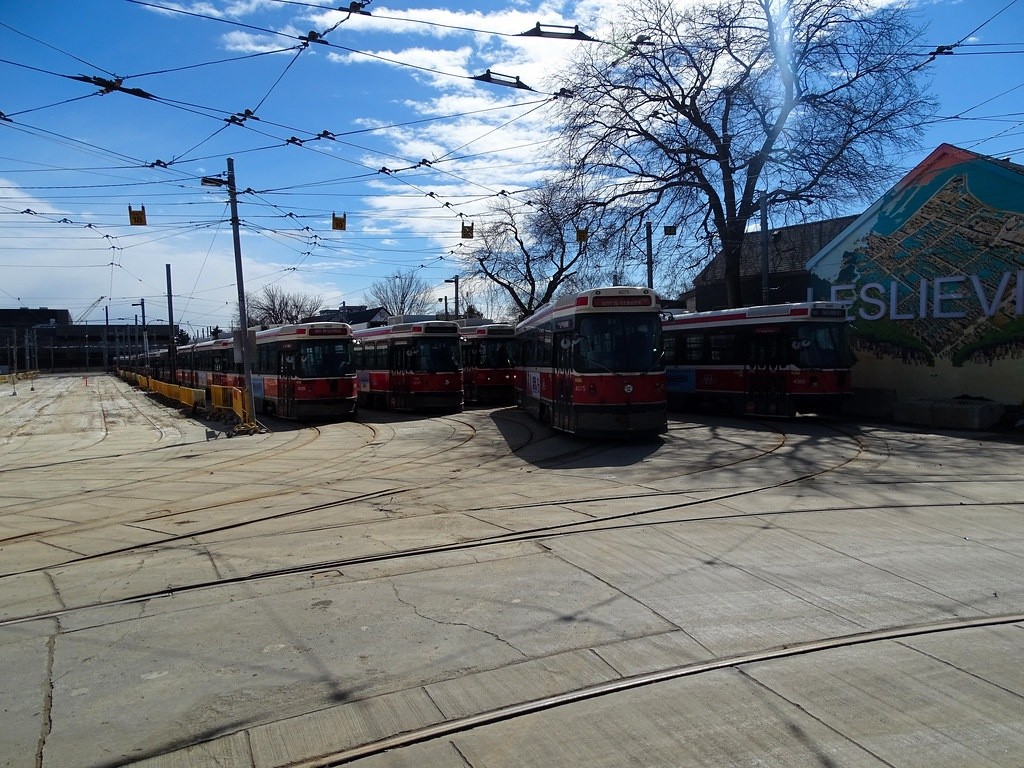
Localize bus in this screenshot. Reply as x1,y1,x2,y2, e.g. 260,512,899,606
515,285,670,440
114,319,359,422
307,320,515,415
662,299,858,415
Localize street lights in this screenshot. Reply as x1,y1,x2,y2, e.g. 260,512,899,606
119,318,164,368
